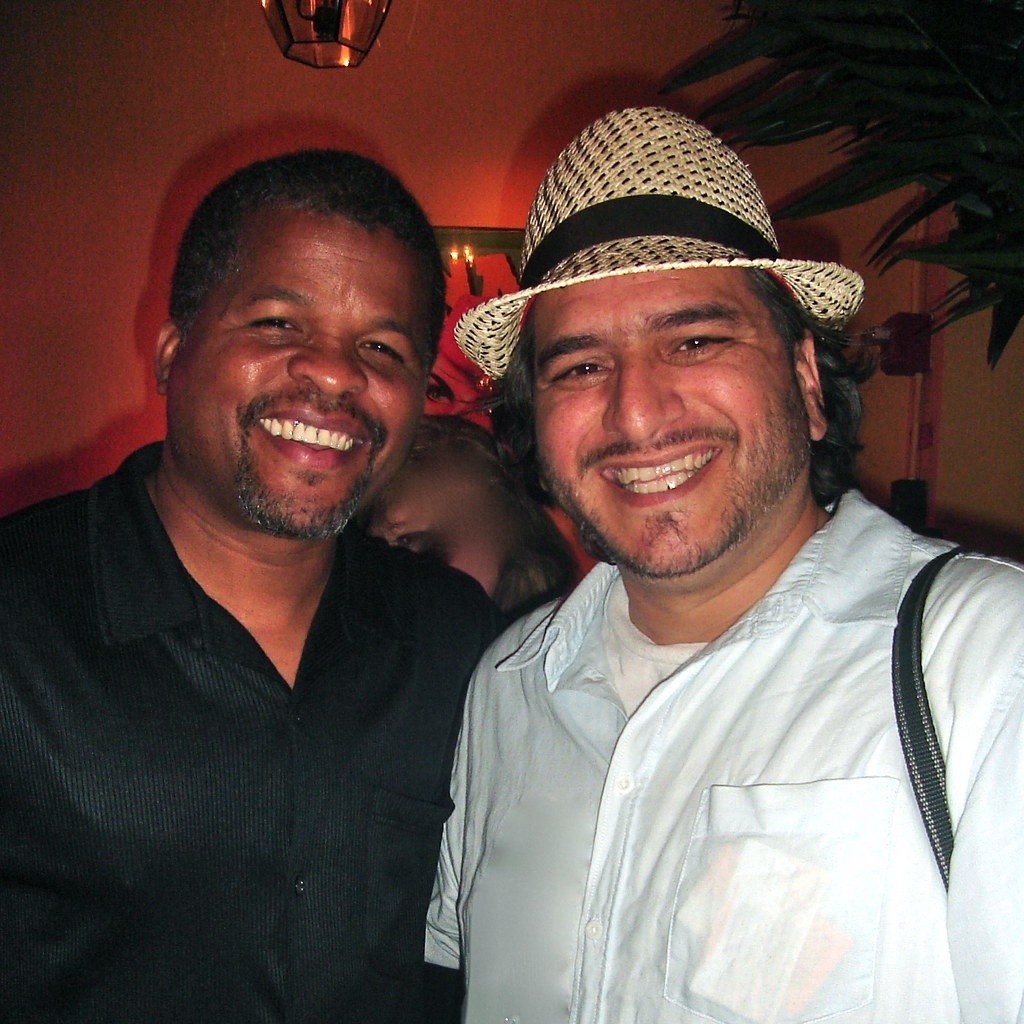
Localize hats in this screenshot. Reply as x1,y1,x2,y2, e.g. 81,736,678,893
452,107,865,383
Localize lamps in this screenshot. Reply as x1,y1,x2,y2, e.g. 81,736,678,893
260,0,393,69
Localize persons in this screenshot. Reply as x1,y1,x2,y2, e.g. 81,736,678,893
417,102,1021,1020
0,144,503,1022
365,411,589,629
